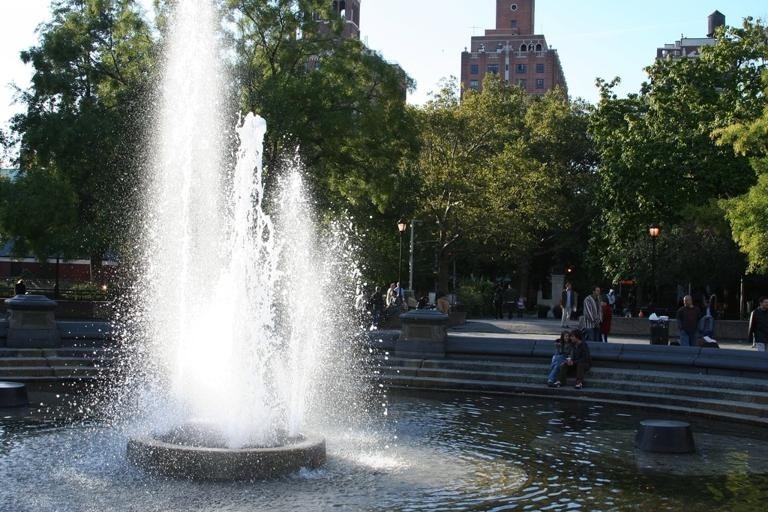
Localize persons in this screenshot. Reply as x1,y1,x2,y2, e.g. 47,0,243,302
357,280,405,326
415,296,429,311
567,329,592,389
558,280,578,331
598,297,613,341
745,295,768,353
547,331,574,389
675,295,701,346
495,288,529,320
582,286,602,342
604,287,616,314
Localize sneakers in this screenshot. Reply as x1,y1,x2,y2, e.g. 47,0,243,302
576,381,585,389
547,379,562,388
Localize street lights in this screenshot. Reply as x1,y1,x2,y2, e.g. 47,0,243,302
397,215,408,297
649,221,661,304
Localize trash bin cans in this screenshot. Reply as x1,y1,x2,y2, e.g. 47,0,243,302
648,319,670,344
